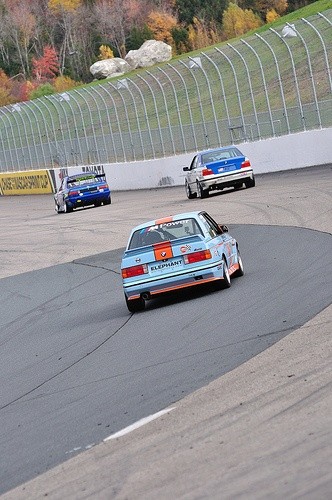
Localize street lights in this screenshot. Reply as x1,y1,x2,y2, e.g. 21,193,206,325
117,78,146,161
9,104,34,169
269,23,306,131
188,55,222,147
58,92,85,166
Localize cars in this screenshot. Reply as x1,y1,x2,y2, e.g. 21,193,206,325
54,172,111,214
121,210,245,314
183,146,254,199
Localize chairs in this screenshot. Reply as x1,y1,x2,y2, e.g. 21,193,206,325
205,159,214,164
146,231,162,245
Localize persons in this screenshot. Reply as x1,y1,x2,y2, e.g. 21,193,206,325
144,228,177,245
